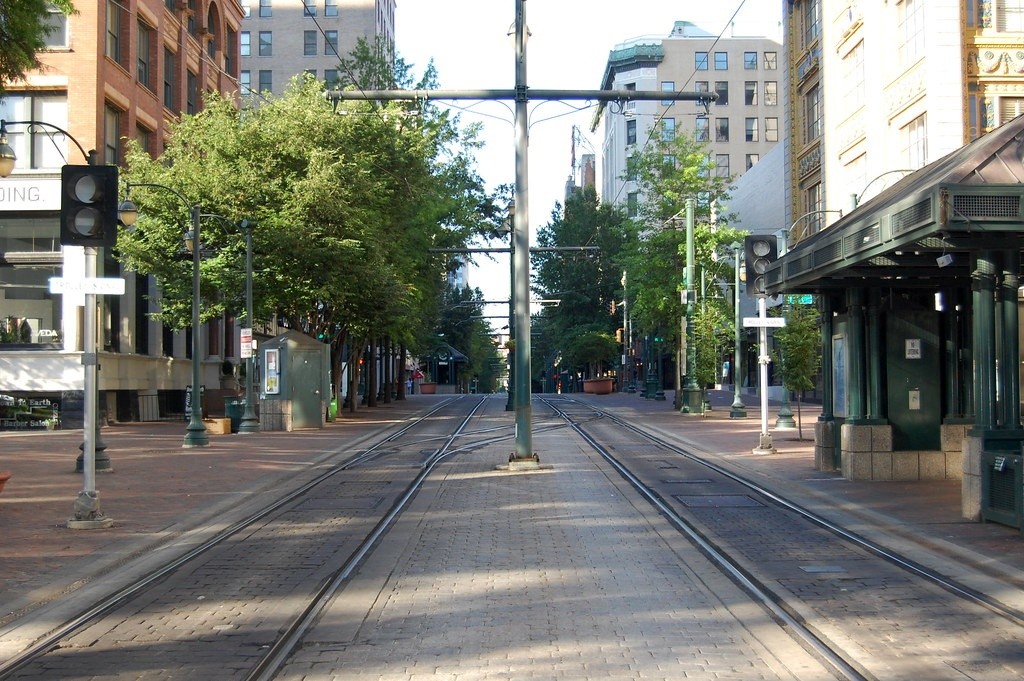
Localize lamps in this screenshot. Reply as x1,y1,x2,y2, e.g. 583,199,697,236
936,237,953,268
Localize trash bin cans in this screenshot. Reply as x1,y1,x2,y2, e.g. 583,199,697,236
326,384,337,422
222,395,241,433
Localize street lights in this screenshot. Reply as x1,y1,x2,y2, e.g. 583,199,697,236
707,285,748,416
120,181,209,448
0,119,110,471
184,212,260,432
743,232,797,428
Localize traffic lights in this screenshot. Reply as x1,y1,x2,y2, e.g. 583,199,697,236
318,326,323,340
59,164,118,246
744,235,777,299
609,300,615,315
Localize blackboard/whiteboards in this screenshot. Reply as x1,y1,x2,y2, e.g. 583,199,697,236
184,384,205,418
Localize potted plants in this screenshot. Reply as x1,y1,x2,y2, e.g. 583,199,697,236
419,372,437,394
562,324,621,395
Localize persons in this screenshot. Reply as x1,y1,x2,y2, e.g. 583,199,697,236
394,376,398,385
404,378,414,394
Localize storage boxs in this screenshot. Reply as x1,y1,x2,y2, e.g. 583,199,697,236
202,418,231,435
